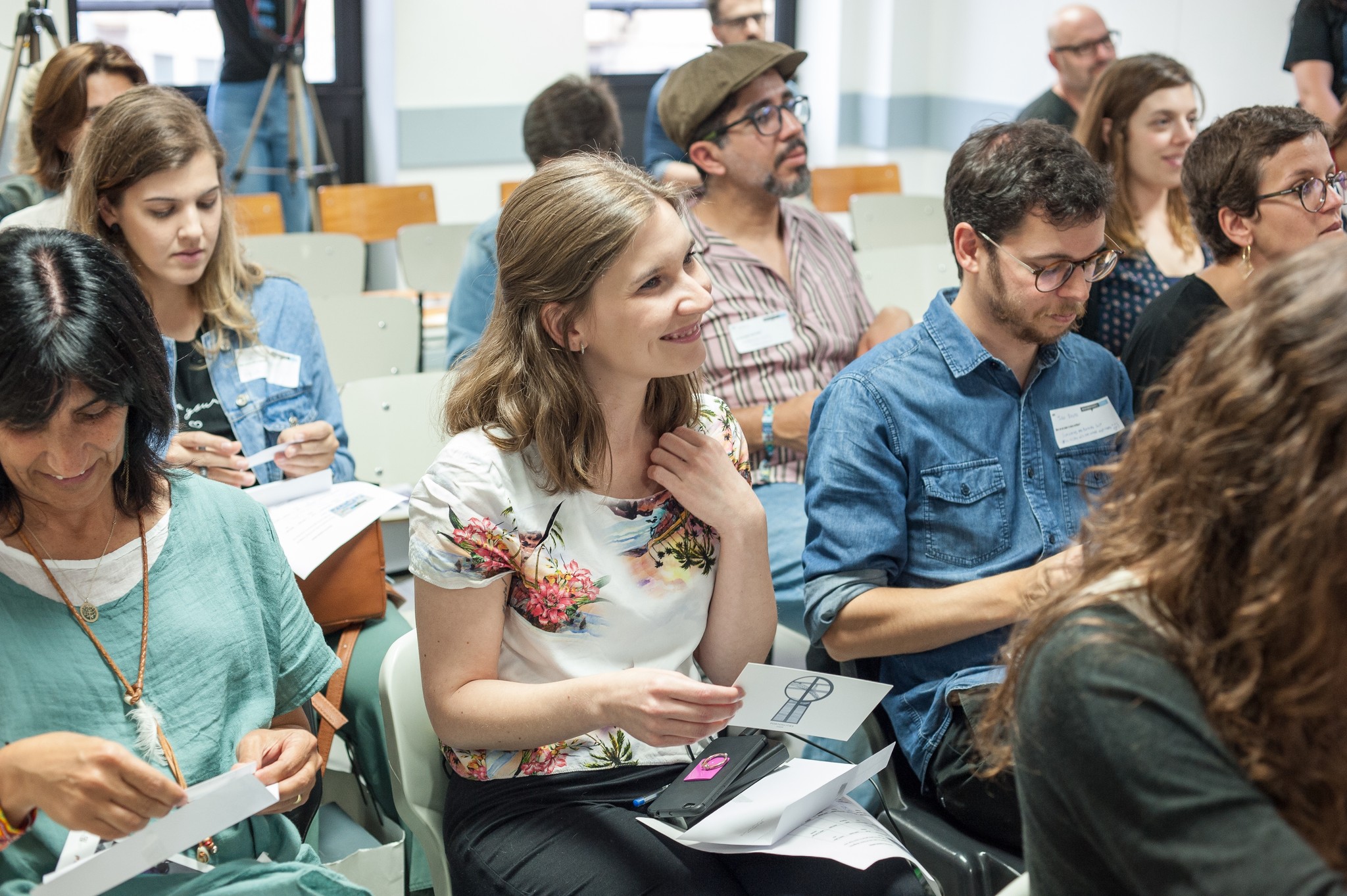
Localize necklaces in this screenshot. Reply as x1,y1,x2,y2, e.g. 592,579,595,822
5,486,221,870
15,501,119,623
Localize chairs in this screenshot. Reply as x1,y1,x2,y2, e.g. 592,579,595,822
232,166,1024,896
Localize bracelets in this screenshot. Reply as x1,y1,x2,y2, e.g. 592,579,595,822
760,402,775,457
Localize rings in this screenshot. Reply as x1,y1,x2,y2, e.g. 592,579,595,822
293,794,302,805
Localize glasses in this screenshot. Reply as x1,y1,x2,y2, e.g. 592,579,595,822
715,12,767,28
701,94,810,142
1054,30,1120,58
1256,170,1347,213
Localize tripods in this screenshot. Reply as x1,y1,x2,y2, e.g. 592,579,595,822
0,0,63,154
232,0,339,236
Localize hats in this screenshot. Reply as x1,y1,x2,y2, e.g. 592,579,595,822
658,39,808,151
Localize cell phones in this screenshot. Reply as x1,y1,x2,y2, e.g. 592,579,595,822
648,735,769,818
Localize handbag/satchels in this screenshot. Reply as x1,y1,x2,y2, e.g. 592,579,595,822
291,516,390,632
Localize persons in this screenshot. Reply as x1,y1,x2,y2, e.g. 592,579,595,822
0,0,315,238
0,224,328,896
654,37,914,638
1019,0,1347,417
975,237,1347,896
445,73,627,368
793,115,1142,846
407,152,930,894
641,0,767,193
66,84,355,486
